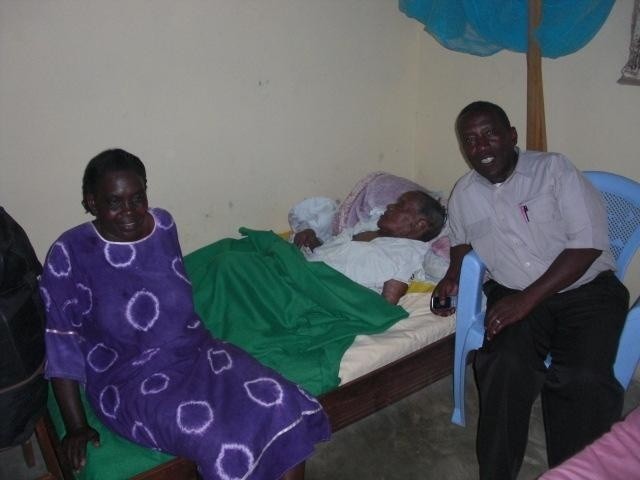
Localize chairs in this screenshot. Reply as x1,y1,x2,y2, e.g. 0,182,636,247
451,167,640,428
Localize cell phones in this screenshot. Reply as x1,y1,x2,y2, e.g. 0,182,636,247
431,296,458,310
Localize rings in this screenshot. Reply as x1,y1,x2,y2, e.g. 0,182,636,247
495,319,501,325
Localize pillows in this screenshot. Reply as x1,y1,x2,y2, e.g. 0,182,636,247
334,170,456,261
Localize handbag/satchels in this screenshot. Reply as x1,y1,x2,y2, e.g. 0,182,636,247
0,207,47,449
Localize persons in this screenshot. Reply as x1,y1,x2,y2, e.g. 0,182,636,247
428,101,630,478
292,189,449,305
33,145,334,480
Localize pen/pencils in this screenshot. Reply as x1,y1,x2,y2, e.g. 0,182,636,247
521,206,529,223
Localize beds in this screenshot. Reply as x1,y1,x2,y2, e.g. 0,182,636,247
22,196,486,479
